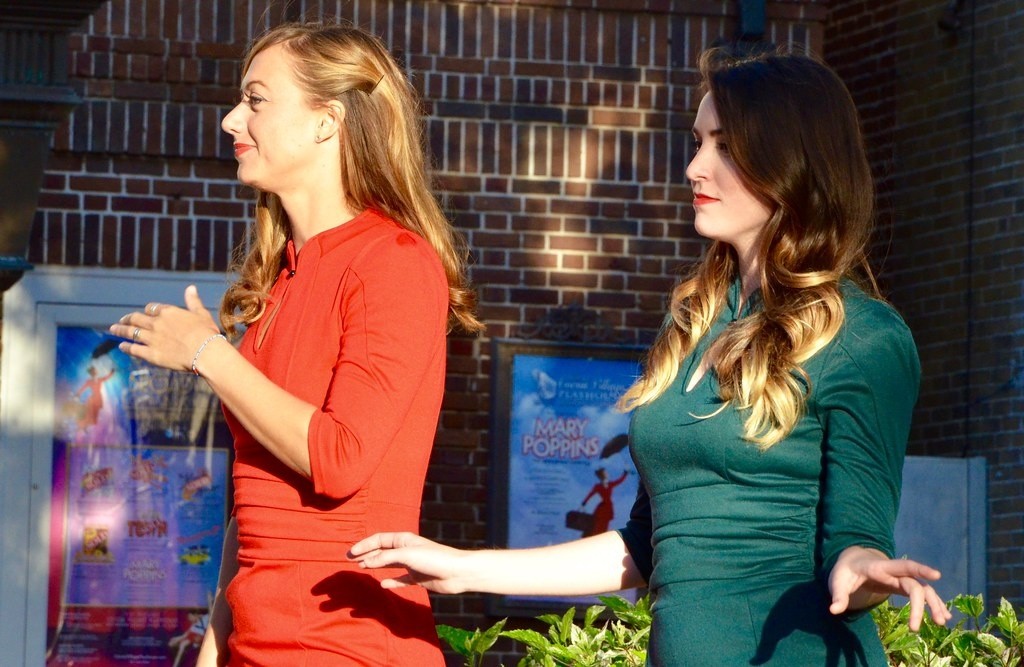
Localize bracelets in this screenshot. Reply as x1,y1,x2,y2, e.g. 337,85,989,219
192,334,226,376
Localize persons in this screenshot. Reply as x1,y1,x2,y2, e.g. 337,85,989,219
111,23,485,667
346,49,952,667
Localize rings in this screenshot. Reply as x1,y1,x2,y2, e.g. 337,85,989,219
132,326,140,342
150,303,160,316
